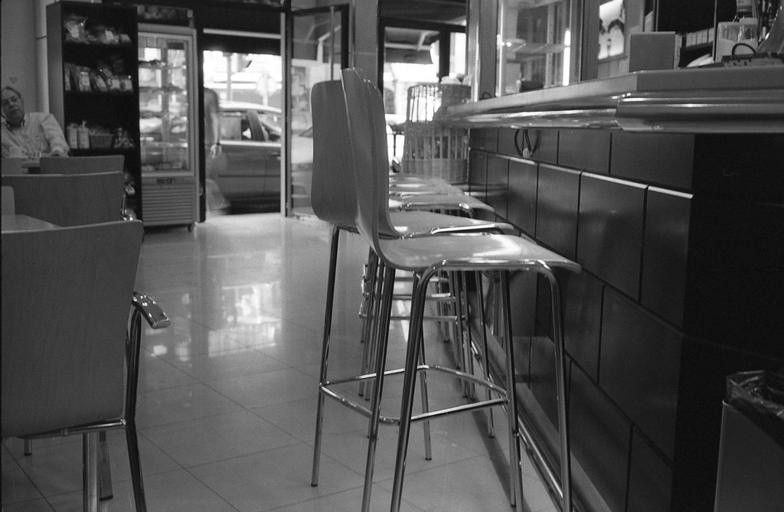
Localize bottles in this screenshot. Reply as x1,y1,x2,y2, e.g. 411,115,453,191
63,66,132,93
714,17,761,63
731,1,777,21
65,119,90,152
114,127,133,149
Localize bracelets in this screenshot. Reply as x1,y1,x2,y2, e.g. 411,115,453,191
216,142,221,145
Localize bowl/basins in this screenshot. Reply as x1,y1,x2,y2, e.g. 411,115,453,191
89,134,113,149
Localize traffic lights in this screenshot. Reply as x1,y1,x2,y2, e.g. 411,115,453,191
237,51,252,73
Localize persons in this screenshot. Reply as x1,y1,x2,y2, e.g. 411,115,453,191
0,86,71,158
203,88,231,210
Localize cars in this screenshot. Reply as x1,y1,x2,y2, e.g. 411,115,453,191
290,113,405,208
139,101,283,204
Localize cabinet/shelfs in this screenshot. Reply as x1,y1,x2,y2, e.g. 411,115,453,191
33,0,144,238
135,20,200,232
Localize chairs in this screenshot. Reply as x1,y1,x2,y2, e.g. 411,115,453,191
0,151,169,512
307,79,514,512
340,67,582,512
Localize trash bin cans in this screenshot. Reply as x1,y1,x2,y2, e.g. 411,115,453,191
713,369,784,512
